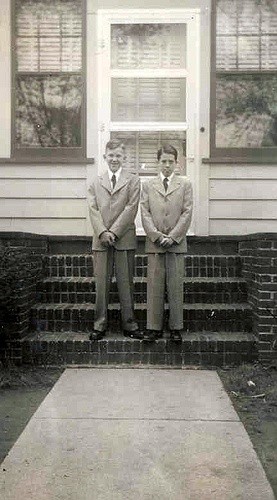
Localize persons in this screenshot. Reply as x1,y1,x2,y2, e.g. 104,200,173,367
86,139,146,341
140,143,194,343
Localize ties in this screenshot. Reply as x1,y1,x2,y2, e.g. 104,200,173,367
111,175,116,190
163,178,169,193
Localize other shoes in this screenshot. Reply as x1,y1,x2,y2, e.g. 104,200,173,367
123,329,144,339
170,330,183,342
143,330,163,342
88,330,106,341
